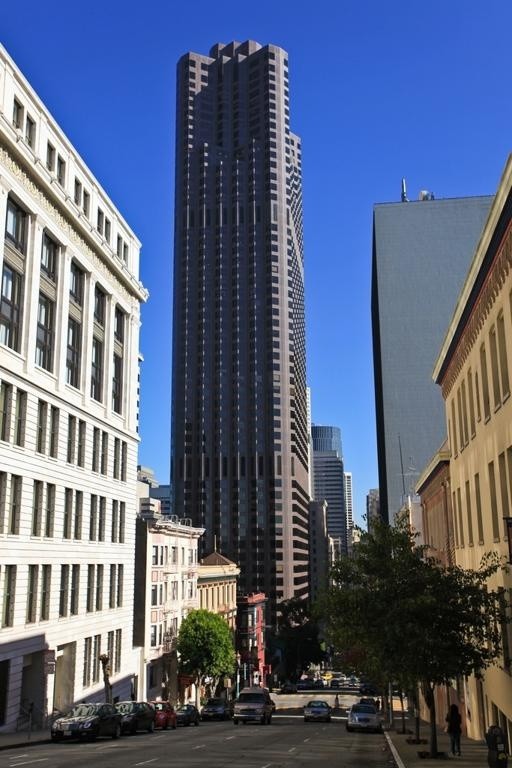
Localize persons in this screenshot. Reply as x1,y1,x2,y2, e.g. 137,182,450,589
485,726,509,768
376,698,380,711
446,704,461,756
334,693,339,709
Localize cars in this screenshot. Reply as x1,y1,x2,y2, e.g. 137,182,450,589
346,698,381,733
50,698,235,743
280,670,364,694
303,701,332,723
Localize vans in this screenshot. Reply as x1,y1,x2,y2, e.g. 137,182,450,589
233,687,273,726
